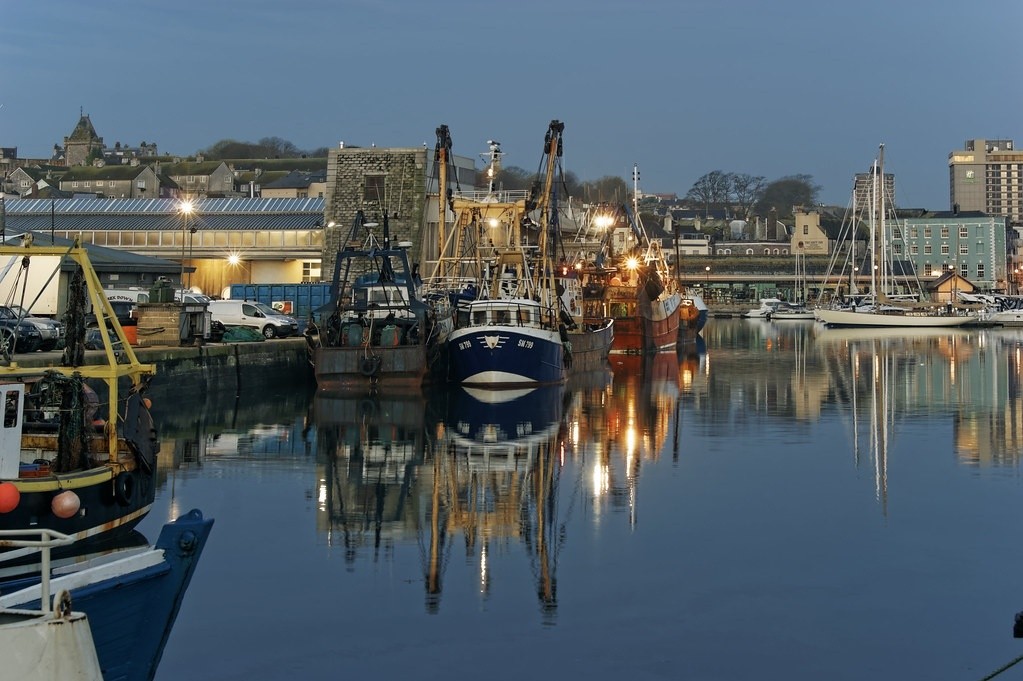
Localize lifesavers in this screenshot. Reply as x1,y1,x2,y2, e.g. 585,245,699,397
359,358,377,376
116,471,137,506
358,399,376,416
559,324,567,342
560,311,571,325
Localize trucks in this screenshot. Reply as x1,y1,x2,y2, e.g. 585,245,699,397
98,287,212,314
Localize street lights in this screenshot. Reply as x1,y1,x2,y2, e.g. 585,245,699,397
180,202,192,306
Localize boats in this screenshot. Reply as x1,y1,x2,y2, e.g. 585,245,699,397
0,243,161,560
1,507,215,681
415,373,572,631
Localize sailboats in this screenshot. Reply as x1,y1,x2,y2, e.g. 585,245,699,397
302,116,709,390
737,141,1023,329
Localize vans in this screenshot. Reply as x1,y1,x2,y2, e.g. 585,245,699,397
206,299,300,340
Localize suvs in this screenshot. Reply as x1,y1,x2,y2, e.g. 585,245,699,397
0,304,61,356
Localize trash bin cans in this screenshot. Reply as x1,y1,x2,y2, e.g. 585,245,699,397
736,291,745,299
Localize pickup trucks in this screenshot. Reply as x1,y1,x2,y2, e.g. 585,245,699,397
86,299,137,328
